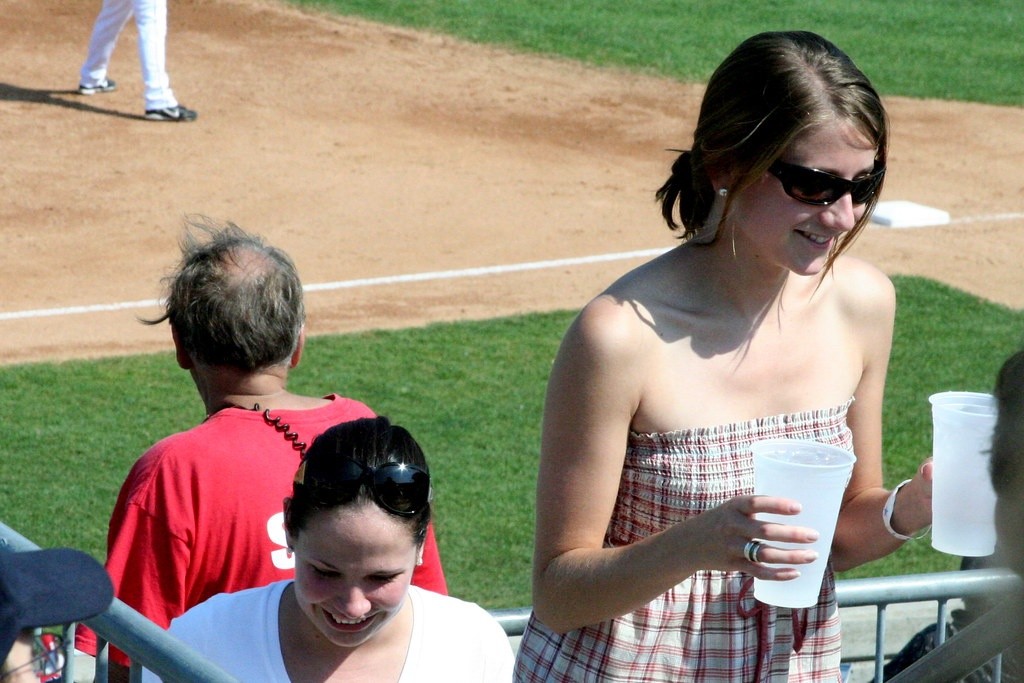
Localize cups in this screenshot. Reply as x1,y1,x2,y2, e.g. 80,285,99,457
928,391,999,556
748,439,857,608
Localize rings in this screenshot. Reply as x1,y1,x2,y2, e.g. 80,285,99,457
744,541,762,562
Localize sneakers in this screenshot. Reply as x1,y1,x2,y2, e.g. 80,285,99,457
145,104,198,122
79,76,117,95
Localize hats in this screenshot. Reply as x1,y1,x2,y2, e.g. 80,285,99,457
0,544,115,666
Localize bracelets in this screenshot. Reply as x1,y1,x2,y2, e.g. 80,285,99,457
882,479,932,541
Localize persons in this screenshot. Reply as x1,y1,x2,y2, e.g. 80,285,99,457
78,0,198,122
142,416,515,683
0,543,115,683
513,31,934,683
75,214,448,683
868,350,1024,683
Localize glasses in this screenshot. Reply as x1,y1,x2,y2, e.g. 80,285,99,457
296,452,432,518
768,158,887,206
0,632,72,680
